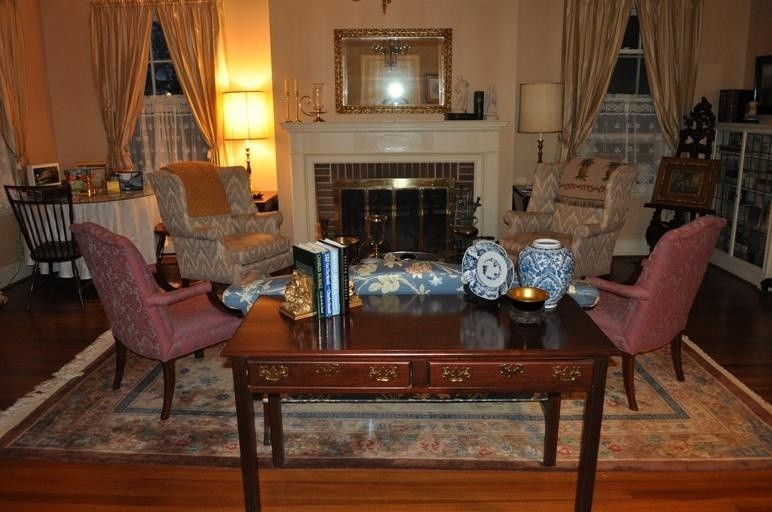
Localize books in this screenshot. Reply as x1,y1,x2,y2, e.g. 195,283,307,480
292,238,349,318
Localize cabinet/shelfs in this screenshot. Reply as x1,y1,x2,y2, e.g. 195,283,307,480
250,191,278,213
695,123,772,293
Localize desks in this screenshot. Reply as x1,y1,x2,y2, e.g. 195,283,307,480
512,184,532,211
218,294,620,512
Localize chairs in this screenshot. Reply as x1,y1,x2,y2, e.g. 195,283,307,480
500,157,640,277
571,215,728,412
69,222,244,420
154,223,180,282
147,161,294,287
3,180,84,316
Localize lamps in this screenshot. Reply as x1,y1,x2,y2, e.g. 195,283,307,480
222,91,269,189
372,40,411,73
517,84,563,165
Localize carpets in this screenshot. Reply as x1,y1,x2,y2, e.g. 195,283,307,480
0,282,772,470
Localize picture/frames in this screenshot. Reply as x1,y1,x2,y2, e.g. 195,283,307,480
651,156,721,208
118,171,143,191
26,162,61,187
754,54,772,115
423,73,439,103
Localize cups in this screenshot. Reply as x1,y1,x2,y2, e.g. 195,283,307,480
106,180,120,194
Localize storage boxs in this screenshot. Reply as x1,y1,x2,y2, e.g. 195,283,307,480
717,89,759,122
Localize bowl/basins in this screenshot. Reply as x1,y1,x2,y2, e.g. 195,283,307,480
507,286,548,311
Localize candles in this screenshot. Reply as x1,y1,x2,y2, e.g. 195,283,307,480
294,79,298,96
283,79,289,98
312,83,322,110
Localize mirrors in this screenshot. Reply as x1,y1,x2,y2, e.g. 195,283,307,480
334,28,452,113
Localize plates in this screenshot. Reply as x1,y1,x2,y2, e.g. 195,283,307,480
462,240,515,301
